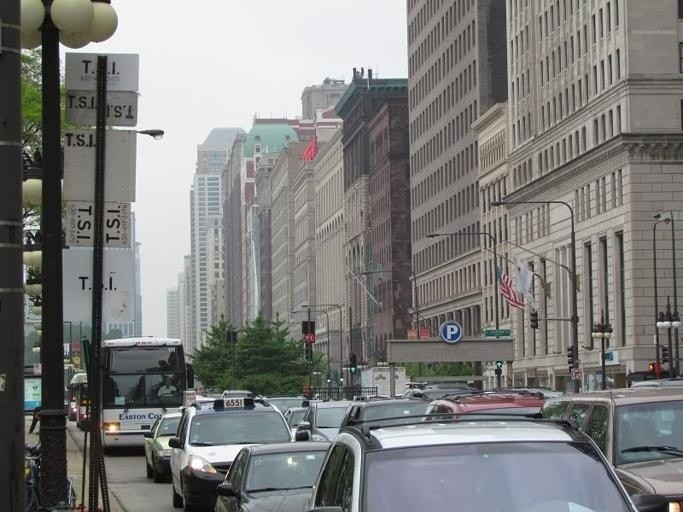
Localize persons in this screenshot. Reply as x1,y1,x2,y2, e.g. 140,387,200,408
157,374,177,398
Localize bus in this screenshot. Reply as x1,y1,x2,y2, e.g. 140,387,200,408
100,335,193,450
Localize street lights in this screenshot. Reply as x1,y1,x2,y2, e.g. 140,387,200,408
651,209,680,382
20,146,44,355
18,0,118,510
487,200,579,394
360,270,421,380
301,304,343,388
591,309,612,391
291,310,330,390
137,129,165,141
424,232,501,390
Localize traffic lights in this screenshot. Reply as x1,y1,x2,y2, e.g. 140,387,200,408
348,353,356,375
495,361,501,369
326,378,330,384
529,311,538,330
338,377,342,387
566,345,574,373
661,346,668,364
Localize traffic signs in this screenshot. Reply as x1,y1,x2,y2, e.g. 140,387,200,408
485,330,510,336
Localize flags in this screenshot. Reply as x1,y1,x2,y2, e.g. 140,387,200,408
514,256,534,300
497,266,526,311
